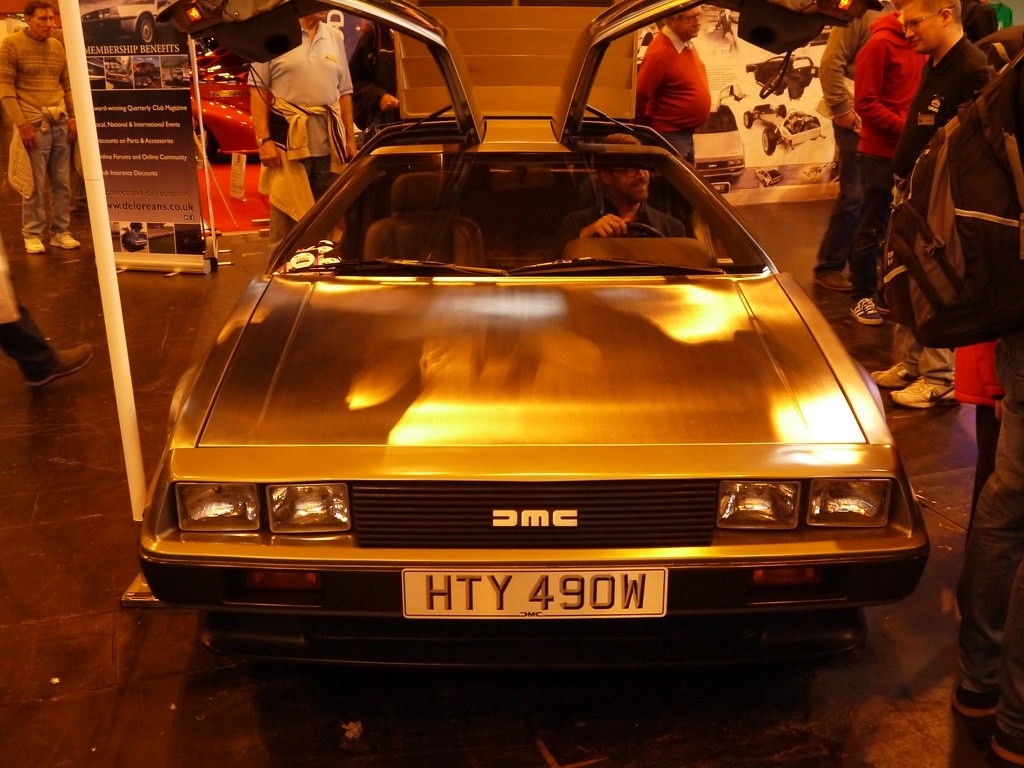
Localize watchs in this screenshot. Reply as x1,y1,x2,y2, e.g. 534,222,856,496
256,136,272,148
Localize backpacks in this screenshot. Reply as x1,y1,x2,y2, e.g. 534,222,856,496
882,44,1024,349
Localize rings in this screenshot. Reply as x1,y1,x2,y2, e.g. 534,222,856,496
609,219,614,226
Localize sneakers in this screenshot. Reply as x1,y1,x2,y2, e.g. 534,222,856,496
50,230,80,250
851,296,884,325
889,377,961,408
874,299,889,313
23,233,45,253
26,342,95,388
870,365,919,389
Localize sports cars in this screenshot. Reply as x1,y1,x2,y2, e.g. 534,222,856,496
188,65,261,166
77,0,183,45
114,0,933,699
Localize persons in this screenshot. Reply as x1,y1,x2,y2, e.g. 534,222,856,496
849,8,930,325
554,133,689,258
871,0,1024,768
348,18,399,131
0,0,84,253
248,7,358,271
635,6,712,158
810,0,898,292
0,237,94,387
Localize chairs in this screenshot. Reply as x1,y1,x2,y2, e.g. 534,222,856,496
361,172,486,267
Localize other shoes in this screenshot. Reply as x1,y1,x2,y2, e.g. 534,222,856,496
991,726,1024,765
951,680,999,716
814,270,852,291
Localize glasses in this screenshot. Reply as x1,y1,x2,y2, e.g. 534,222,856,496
901,3,955,33
609,168,639,178
